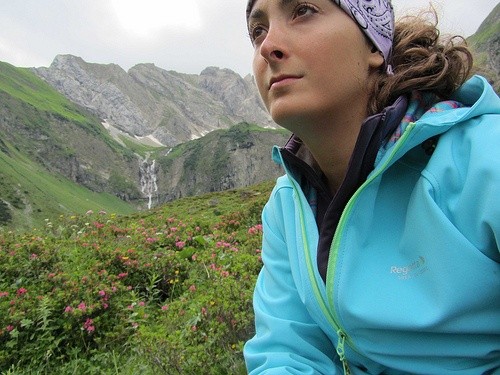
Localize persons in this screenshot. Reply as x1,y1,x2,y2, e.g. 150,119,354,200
245,0,500,375
